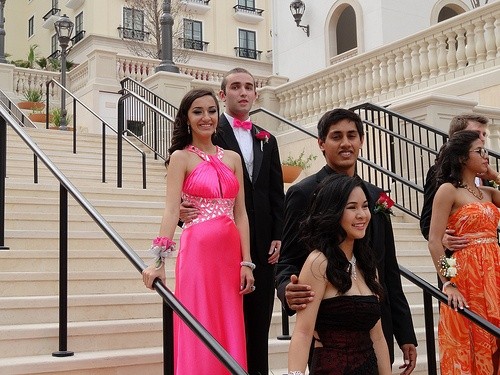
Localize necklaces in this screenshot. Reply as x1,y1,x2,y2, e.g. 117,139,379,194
347,255,357,280
459,181,484,200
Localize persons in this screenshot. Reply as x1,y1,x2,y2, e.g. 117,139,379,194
276,108,418,375
287,173,393,375
420,112,500,375
177,67,285,375
142,88,257,375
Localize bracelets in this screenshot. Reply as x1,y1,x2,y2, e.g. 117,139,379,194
151,236,176,269
444,280,456,289
240,262,256,269
493,173,500,184
288,371,305,375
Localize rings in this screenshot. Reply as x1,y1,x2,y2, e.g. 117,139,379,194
251,286,255,291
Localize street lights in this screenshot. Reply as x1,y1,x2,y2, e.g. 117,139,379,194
53,13,74,130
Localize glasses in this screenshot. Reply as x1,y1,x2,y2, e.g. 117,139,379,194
468,148,488,158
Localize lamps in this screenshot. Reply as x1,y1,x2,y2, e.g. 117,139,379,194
290,0,309,37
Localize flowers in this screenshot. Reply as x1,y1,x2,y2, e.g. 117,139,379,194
256,131,270,144
374,192,394,213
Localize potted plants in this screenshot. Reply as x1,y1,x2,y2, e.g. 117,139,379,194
281,148,317,183
17,88,45,111
29,100,53,123
47,107,73,131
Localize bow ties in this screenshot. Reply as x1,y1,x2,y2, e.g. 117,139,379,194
233,118,252,131
